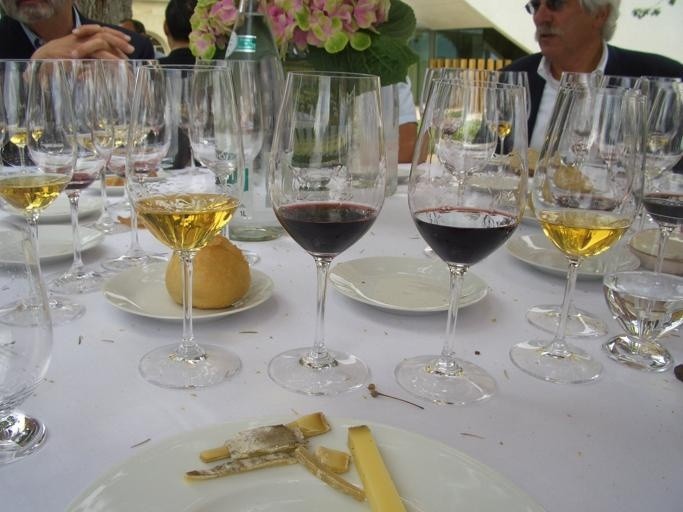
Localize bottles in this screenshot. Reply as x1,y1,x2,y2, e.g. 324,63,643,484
211,0,288,245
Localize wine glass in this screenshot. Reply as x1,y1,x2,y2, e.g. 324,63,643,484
92,60,172,273
419,67,532,120
394,79,527,407
540,72,648,164
125,64,242,388
1,59,84,328
510,87,646,384
634,78,683,190
524,88,637,339
267,69,388,396
26,58,113,295
190,61,264,267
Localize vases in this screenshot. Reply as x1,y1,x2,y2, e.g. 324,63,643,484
257,74,370,171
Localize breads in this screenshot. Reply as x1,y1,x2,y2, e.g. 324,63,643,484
166,236,249,310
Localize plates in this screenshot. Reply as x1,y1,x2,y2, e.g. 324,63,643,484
505,232,641,282
466,177,523,195
395,167,426,185
105,262,274,319
65,412,545,512
331,256,486,317
90,176,126,196
2,193,104,224
0,226,105,267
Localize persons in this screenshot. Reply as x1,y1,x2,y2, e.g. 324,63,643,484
472,0,683,174
154,0,201,78
1,1,194,169
112,16,147,39
352,76,430,166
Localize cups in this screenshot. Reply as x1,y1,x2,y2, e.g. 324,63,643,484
605,195,683,372
0,211,53,464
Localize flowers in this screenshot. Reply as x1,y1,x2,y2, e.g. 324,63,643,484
189,9,425,86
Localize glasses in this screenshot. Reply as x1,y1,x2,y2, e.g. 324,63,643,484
525,0,571,14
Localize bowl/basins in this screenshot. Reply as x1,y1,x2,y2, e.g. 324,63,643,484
629,229,683,275
